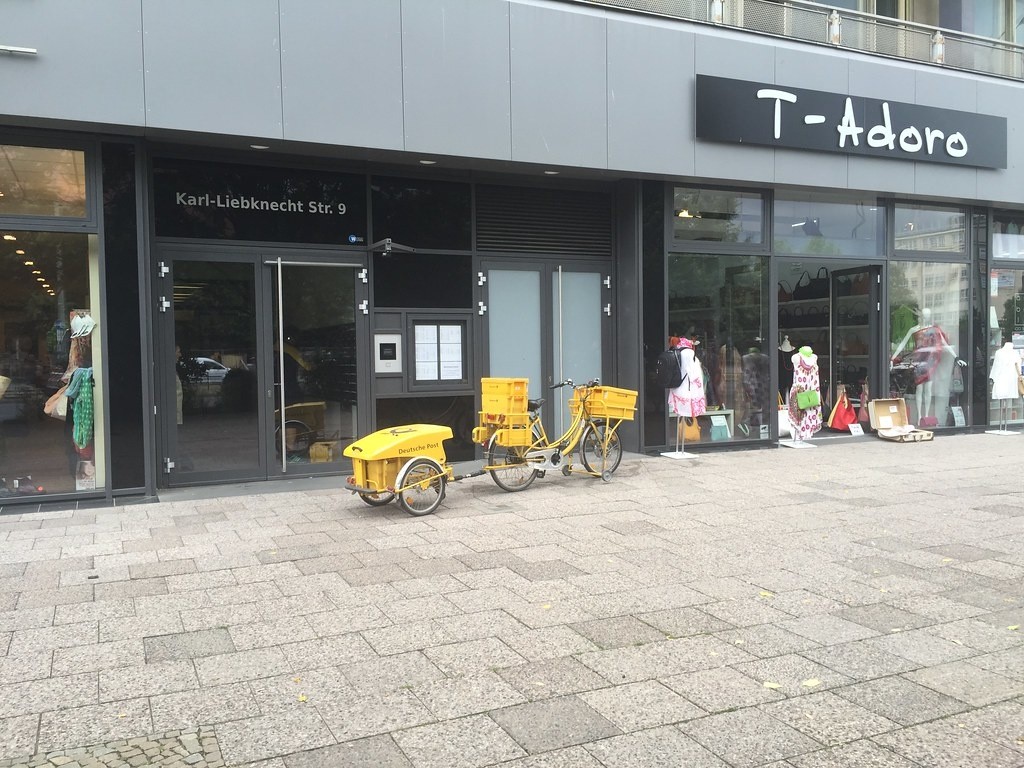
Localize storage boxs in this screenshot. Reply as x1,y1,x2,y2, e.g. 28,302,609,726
573,385,638,418
481,394,529,414
309,441,339,463
352,458,408,490
481,377,529,396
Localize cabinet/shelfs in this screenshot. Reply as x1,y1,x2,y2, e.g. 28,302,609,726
721,294,869,408
668,305,719,343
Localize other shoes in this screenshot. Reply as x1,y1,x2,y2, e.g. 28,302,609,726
0,476,46,496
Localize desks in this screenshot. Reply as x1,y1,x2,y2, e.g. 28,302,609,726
669,409,735,437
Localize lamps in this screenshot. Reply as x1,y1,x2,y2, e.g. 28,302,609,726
801,217,823,237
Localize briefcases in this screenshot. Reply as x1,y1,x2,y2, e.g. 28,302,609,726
867,397,934,443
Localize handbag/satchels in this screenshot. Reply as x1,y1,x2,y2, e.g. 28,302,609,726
1017,374,1024,397
797,390,819,410
679,417,701,442
920,417,939,427
43,383,68,422
827,389,858,431
950,378,964,393
888,354,918,394
710,424,728,441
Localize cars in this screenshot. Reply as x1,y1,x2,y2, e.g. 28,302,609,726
179,356,232,384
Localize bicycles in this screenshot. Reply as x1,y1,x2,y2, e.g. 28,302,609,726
470,374,640,493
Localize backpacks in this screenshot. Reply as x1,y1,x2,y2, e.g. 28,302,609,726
655,345,696,388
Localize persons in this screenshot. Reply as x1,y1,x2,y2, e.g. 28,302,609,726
174,343,184,426
742,348,768,425
988,341,1022,399
778,340,798,405
890,307,968,426
789,346,823,440
668,337,706,417
